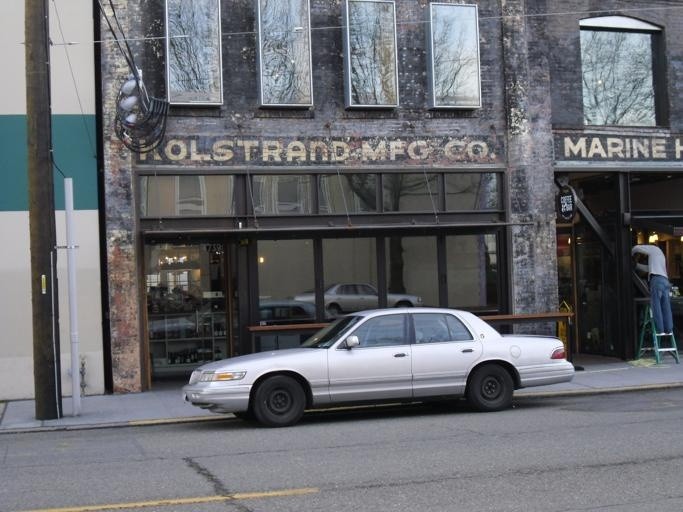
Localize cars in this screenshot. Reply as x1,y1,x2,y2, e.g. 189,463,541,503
147,299,227,344
258,298,341,337
184,307,574,427
293,281,423,317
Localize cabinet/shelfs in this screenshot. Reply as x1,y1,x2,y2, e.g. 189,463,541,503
146,309,229,373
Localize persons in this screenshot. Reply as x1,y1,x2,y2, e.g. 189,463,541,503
632,242,675,335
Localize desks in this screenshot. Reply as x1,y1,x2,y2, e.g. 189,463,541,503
247,311,582,372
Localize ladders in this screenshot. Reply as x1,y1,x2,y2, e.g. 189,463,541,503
636,303,680,364
